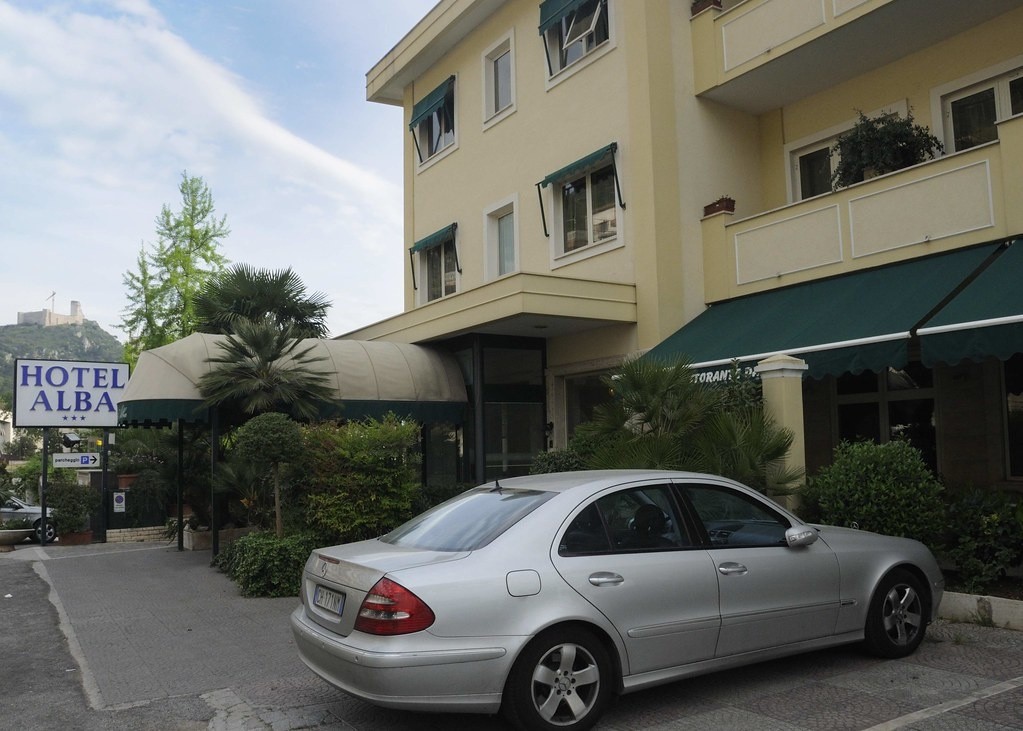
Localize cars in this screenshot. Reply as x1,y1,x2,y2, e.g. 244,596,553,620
289,468,945,731
0,492,61,544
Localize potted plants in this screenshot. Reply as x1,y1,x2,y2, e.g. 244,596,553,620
111,456,141,490
691,0,722,15
703,194,736,216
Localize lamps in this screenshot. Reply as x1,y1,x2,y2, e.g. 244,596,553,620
541,422,554,439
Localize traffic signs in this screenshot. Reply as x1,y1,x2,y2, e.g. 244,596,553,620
52,453,100,468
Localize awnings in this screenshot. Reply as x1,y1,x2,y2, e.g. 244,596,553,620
611,232,1023,383
409,74,456,163
534,142,637,238
538,0,609,76
408,221,466,289
